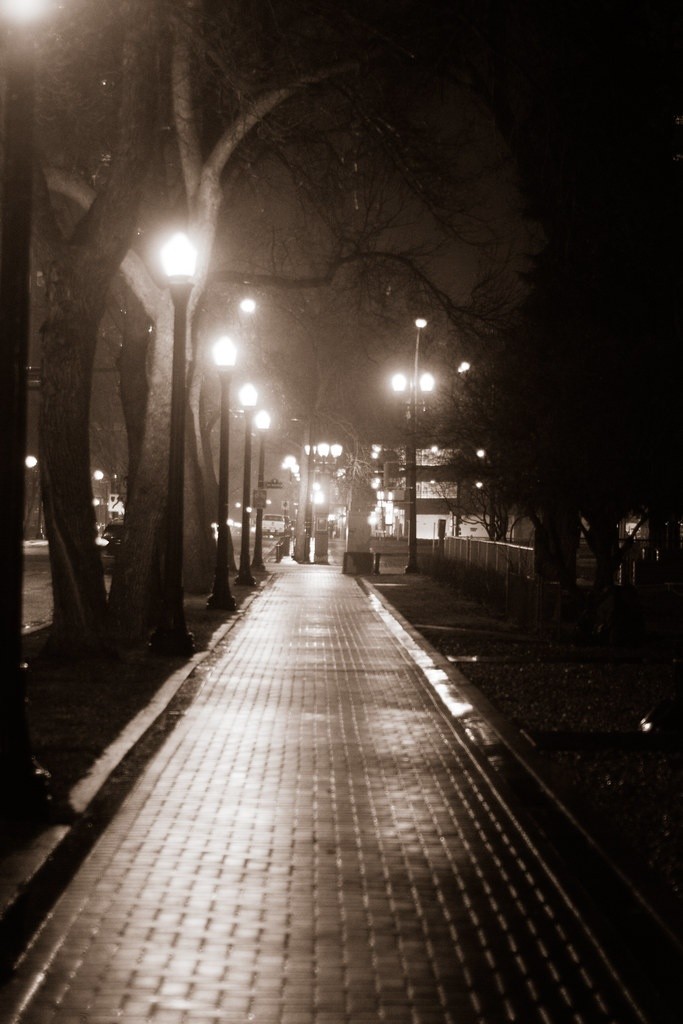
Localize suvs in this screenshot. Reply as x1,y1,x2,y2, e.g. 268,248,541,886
261,513,285,538
98,518,123,576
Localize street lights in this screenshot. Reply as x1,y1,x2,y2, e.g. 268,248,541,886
235,380,258,587
280,454,301,534
391,370,436,575
250,408,269,575
303,444,343,503
414,317,428,413
144,234,201,658
206,334,236,611
